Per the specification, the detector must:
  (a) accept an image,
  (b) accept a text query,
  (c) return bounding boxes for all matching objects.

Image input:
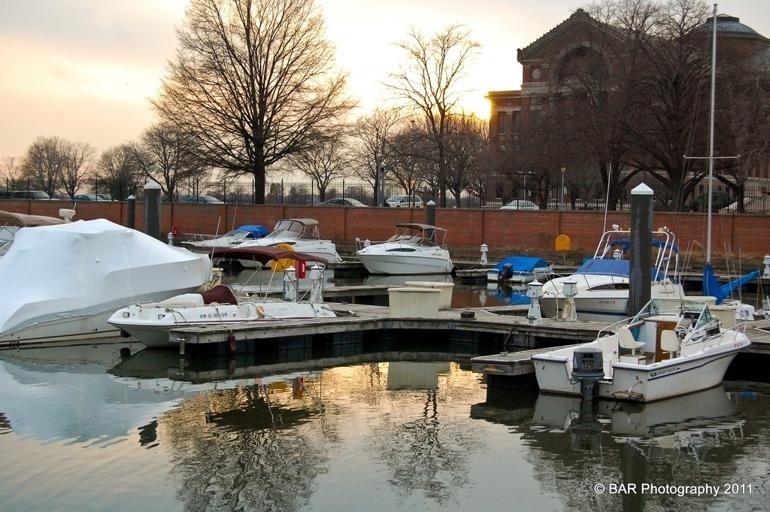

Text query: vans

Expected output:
[384,195,423,209]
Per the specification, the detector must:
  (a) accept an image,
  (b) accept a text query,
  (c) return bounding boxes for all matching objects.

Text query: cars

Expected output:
[317,197,371,208]
[178,195,226,204]
[1,190,113,201]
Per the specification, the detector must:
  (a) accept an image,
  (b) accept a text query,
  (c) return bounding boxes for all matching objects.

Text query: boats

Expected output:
[0,199,454,348]
[487,3,770,405]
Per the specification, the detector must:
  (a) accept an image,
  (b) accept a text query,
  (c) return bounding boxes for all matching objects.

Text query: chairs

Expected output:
[660,330,681,360]
[617,327,646,355]
